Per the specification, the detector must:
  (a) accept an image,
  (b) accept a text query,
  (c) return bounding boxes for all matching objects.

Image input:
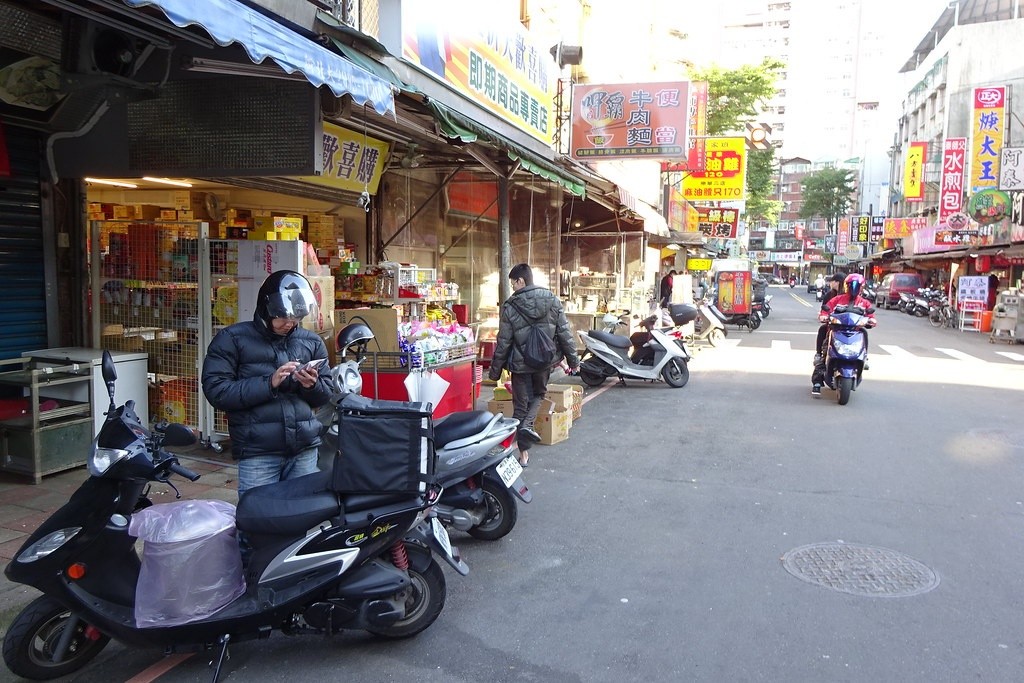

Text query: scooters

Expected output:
[631,257,774,352]
[600,295,699,355]
[313,341,533,542]
[819,304,875,406]
[814,284,828,302]
[896,285,959,329]
[576,311,694,388]
[0,350,472,683]
[789,278,796,289]
[859,279,883,304]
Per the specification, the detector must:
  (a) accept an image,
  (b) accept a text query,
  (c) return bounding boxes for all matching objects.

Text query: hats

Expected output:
[827,272,847,282]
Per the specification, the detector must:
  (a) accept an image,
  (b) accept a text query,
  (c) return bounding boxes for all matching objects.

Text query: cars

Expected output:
[758,272,784,285]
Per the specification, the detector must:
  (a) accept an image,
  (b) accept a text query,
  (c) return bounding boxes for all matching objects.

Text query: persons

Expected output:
[489,264,580,467]
[202,270,334,501]
[790,277,795,283]
[941,279,956,297]
[660,270,678,307]
[811,272,876,395]
[988,274,999,310]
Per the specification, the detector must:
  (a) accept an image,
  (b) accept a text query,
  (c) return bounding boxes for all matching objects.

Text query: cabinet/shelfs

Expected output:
[88,217,240,434]
[959,300,983,333]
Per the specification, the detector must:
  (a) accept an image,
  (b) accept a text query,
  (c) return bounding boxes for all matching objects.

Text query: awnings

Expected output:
[53,0,670,258]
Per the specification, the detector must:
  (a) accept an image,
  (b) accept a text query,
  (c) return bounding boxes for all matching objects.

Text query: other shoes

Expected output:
[864,364,869,370]
[812,386,821,395]
[814,354,821,362]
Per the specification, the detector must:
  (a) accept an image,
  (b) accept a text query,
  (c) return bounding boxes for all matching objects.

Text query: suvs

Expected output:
[874,273,925,310]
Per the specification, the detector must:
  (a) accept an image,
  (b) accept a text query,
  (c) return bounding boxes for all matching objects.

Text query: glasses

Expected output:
[511,279,518,288]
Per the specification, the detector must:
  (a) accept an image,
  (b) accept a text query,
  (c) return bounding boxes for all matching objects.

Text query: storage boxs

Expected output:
[488,382,584,445]
[84,188,458,432]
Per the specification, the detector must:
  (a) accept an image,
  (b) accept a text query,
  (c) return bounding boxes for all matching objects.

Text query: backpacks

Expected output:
[506,300,557,370]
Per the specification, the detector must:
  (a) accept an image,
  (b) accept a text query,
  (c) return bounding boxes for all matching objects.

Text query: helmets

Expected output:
[336,323,375,353]
[257,269,314,321]
[818,274,823,278]
[845,273,864,291]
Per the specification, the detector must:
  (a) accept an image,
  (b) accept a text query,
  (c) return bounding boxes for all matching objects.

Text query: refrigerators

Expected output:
[23,347,148,453]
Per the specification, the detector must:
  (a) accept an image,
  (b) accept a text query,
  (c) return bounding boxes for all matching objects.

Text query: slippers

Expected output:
[519,457,528,467]
[520,428,541,442]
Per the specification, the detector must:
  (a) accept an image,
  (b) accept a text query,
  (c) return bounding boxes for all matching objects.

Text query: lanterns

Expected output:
[975,255,991,272]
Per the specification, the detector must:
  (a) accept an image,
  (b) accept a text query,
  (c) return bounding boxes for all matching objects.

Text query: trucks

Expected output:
[804,261,833,293]
